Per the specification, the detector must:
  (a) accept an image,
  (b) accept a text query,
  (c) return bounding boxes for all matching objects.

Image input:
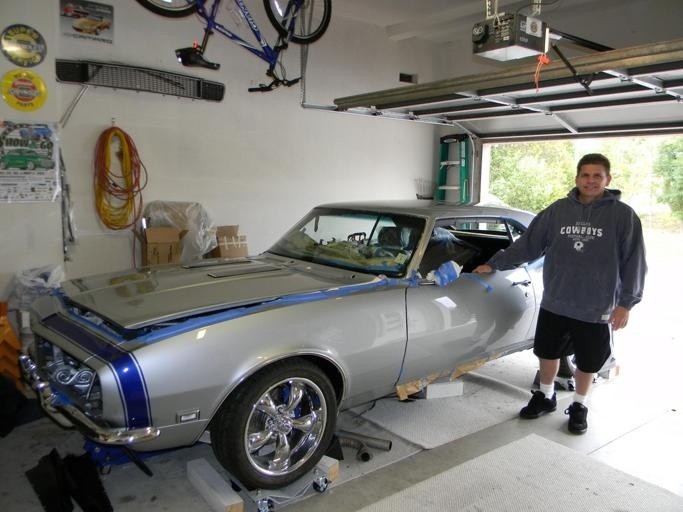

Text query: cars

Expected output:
[0,149,54,171]
[60,3,90,18]
[72,14,112,36]
[15,203,621,491]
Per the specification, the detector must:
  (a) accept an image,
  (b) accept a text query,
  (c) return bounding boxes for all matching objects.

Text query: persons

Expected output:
[470,153,647,437]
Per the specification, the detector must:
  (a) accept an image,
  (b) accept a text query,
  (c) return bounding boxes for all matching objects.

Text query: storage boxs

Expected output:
[210,224,248,257]
[130,226,193,266]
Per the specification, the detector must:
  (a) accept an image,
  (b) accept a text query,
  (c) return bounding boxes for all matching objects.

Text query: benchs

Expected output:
[368,226,511,277]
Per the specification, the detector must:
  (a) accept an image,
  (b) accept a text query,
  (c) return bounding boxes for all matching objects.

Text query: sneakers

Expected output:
[520,390,556,419]
[565,402,588,433]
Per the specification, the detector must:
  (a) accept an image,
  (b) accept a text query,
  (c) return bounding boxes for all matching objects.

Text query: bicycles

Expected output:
[137,0,337,94]
